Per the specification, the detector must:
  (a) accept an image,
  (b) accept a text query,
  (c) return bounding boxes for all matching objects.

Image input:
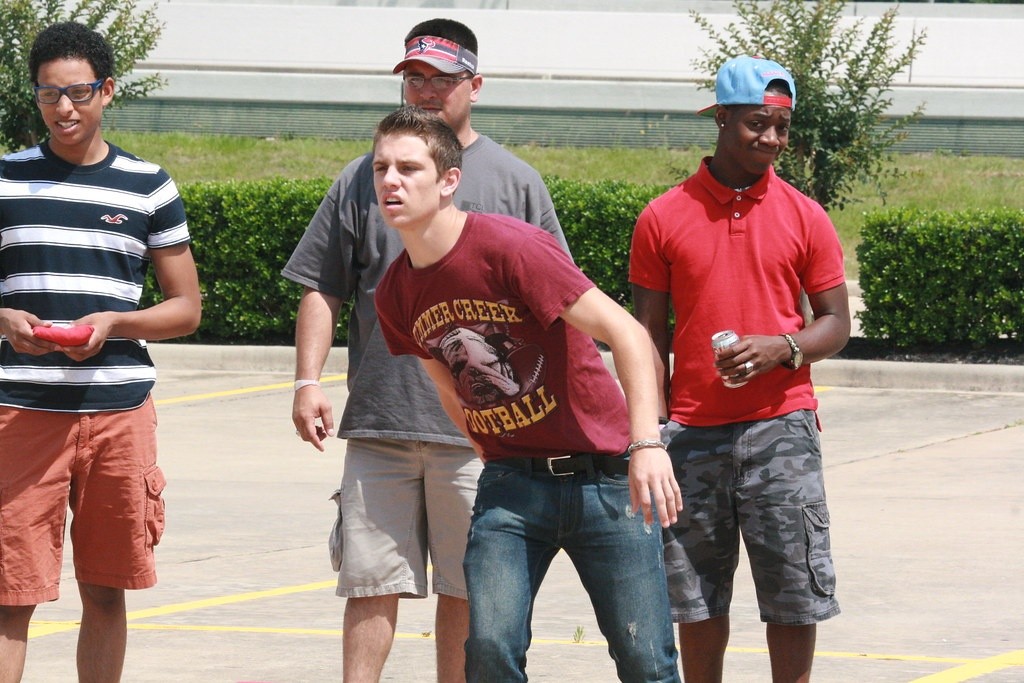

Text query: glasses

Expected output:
[402,74,471,91]
[31,80,101,104]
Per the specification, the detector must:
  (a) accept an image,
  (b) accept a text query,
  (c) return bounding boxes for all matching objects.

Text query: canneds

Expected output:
[711,330,749,388]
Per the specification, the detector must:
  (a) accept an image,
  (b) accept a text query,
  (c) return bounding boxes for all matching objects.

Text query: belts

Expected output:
[502,452,630,477]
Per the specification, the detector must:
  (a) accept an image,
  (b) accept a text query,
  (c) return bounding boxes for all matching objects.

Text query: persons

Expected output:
[0,21,200,683]
[280,19,574,683]
[628,55,857,683]
[371,107,686,683]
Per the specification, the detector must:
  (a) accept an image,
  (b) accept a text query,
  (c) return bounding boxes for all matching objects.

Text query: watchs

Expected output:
[778,332,804,370]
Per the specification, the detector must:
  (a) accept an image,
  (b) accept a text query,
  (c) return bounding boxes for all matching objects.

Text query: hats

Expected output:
[696,54,795,116]
[393,36,478,76]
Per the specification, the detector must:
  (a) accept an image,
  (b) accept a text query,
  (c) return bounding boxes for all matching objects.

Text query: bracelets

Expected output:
[293,380,320,391]
[628,440,667,453]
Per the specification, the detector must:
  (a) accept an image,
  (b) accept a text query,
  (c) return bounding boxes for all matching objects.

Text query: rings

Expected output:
[296,431,301,436]
[745,361,753,374]
